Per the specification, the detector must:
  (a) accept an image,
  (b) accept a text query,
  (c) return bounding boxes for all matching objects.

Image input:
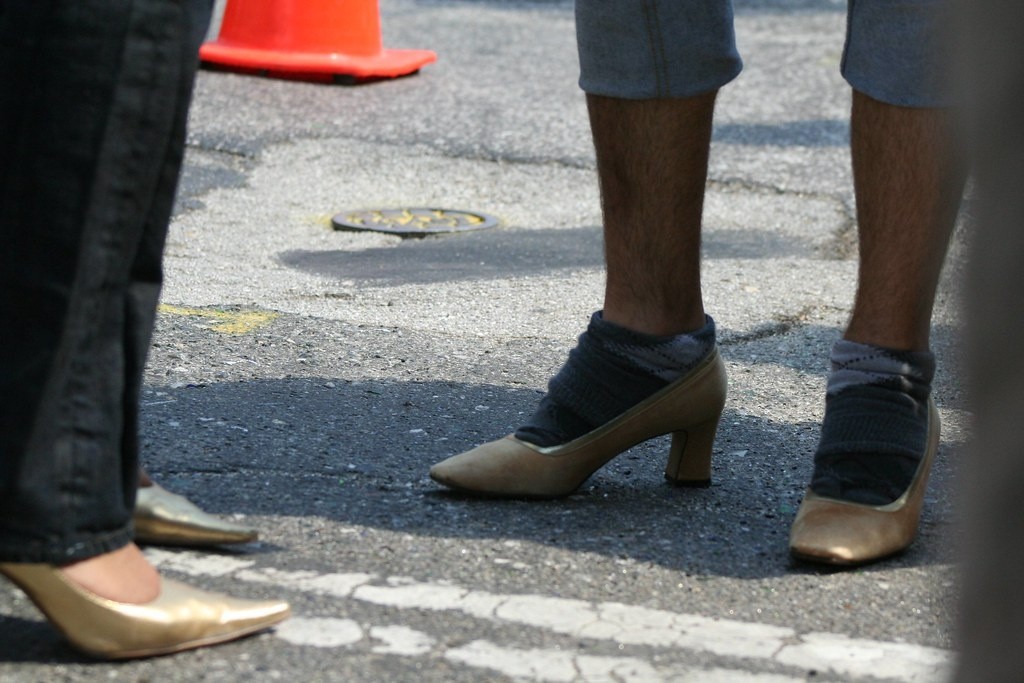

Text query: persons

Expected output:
[431,0,978,567]
[0,0,290,658]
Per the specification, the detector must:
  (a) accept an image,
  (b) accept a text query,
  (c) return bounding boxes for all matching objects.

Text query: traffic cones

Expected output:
[193,0,438,83]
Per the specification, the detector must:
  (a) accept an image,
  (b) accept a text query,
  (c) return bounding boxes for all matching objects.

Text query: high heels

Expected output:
[0,562,291,658]
[428,342,728,495]
[133,480,258,544]
[788,393,942,563]
